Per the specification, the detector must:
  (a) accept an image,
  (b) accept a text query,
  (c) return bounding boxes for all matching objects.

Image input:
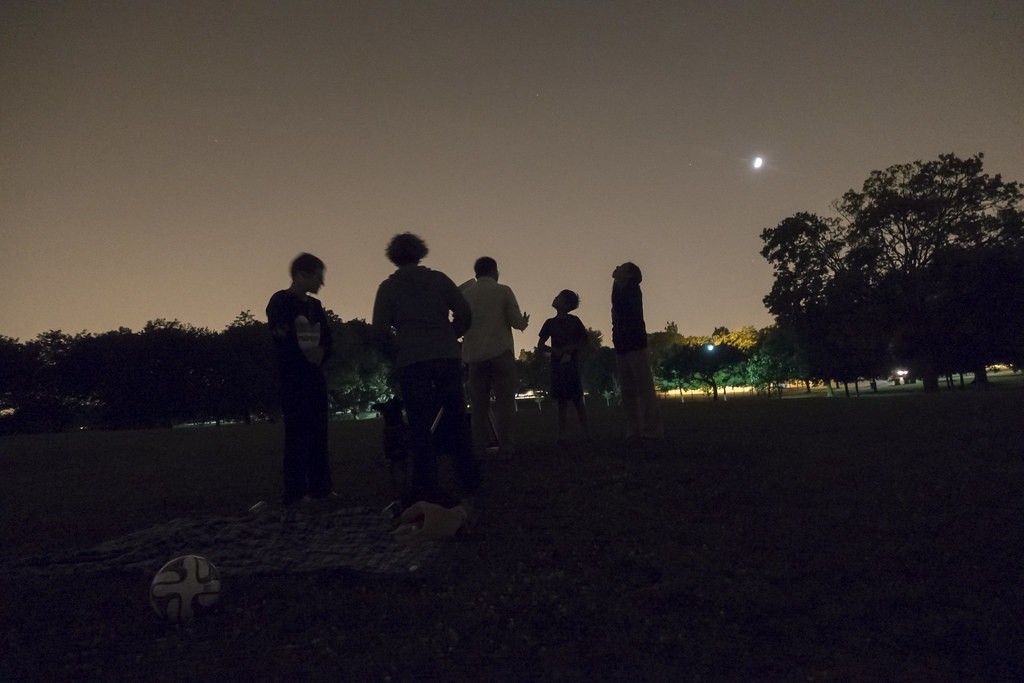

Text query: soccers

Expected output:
[149,556,223,621]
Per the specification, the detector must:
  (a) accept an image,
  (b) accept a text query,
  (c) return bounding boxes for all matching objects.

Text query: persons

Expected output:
[536,290,596,443]
[609,261,659,438]
[371,231,475,490]
[455,254,530,462]
[265,252,340,510]
